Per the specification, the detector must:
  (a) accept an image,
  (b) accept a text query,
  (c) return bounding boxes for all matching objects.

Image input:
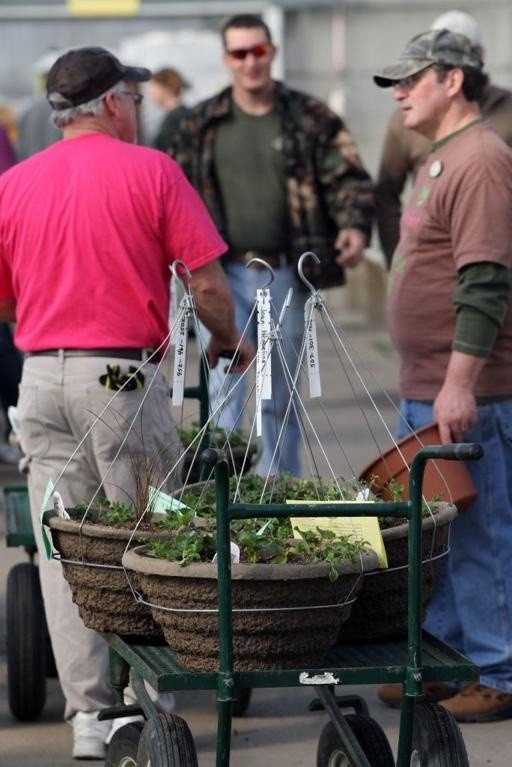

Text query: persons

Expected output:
[154,14,374,481]
[0,123,24,463]
[0,45,255,761]
[145,70,191,143]
[373,29,512,724]
[374,11,512,271]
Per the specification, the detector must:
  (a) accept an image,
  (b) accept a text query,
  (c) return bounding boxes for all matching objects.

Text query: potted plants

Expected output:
[330,476,460,644]
[171,470,327,520]
[44,502,169,640]
[172,416,259,486]
[121,510,376,675]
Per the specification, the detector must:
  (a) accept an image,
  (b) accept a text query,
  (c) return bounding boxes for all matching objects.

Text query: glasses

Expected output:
[223,42,274,61]
[99,84,146,105]
[389,65,455,89]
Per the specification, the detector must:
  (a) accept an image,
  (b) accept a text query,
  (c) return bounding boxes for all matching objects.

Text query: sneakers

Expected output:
[377,681,512,723]
[71,713,147,761]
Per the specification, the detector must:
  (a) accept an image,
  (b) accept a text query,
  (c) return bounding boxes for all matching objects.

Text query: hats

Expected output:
[432,11,482,53]
[371,28,484,90]
[41,44,154,110]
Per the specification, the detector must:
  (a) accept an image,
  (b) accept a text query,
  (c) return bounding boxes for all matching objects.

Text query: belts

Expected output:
[224,247,297,270]
[27,338,162,370]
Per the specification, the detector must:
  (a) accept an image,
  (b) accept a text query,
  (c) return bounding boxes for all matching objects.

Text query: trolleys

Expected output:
[5,436,265,723]
[47,440,491,767]
